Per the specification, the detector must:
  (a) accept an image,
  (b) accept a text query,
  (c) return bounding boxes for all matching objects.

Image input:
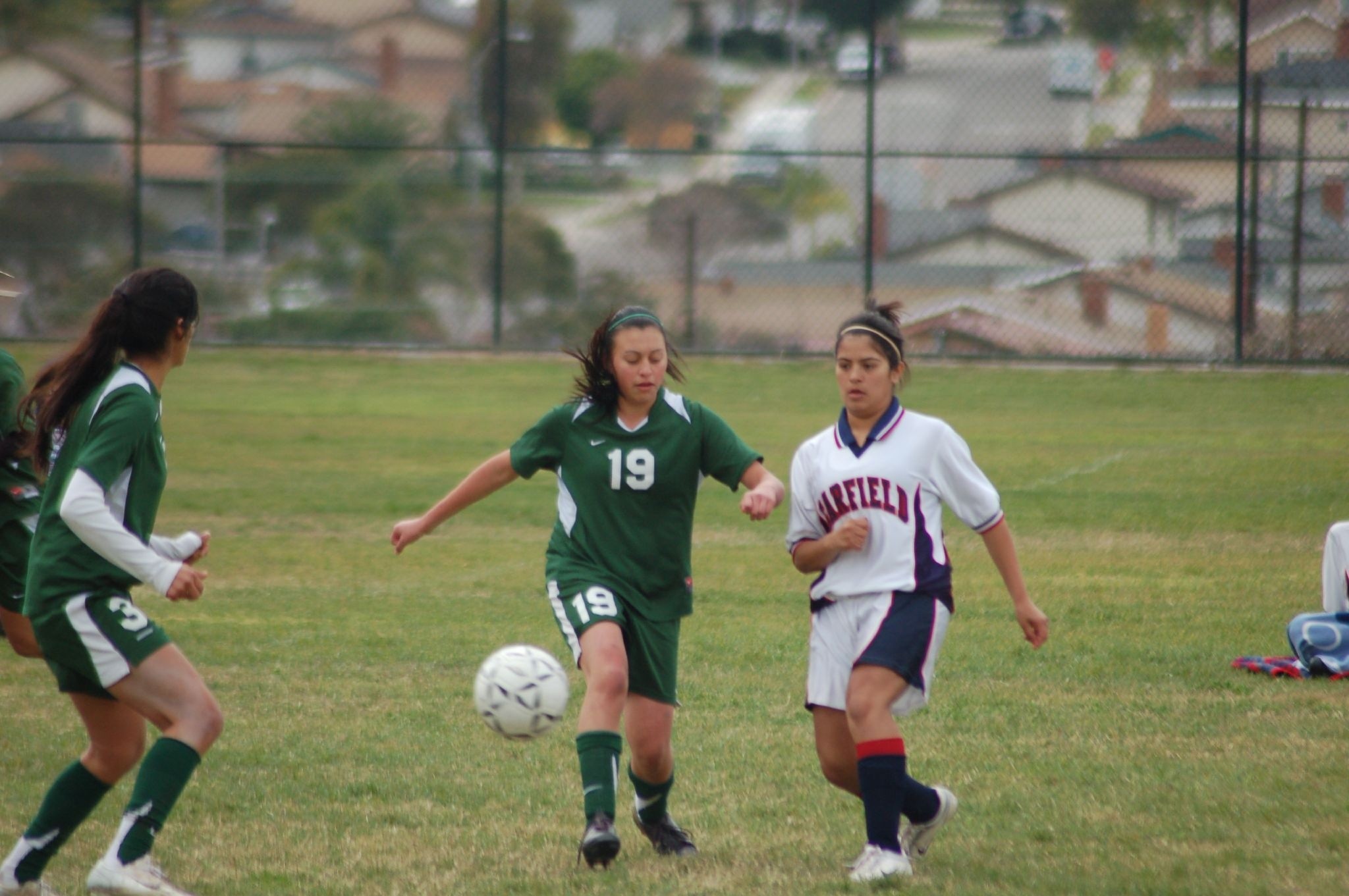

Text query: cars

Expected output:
[831,33,886,85]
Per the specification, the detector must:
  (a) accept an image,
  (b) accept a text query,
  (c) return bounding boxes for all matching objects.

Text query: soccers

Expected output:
[474,644,569,736]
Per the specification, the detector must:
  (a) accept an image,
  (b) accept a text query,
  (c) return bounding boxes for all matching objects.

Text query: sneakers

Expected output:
[86,854,192,896]
[632,806,696,862]
[899,787,958,864]
[0,881,56,896]
[846,843,915,885]
[578,812,620,869]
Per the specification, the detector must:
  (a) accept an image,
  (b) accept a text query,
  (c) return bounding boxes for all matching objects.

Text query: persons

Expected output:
[785,298,1049,882]
[0,349,45,659]
[390,307,785,872]
[0,268,225,893]
